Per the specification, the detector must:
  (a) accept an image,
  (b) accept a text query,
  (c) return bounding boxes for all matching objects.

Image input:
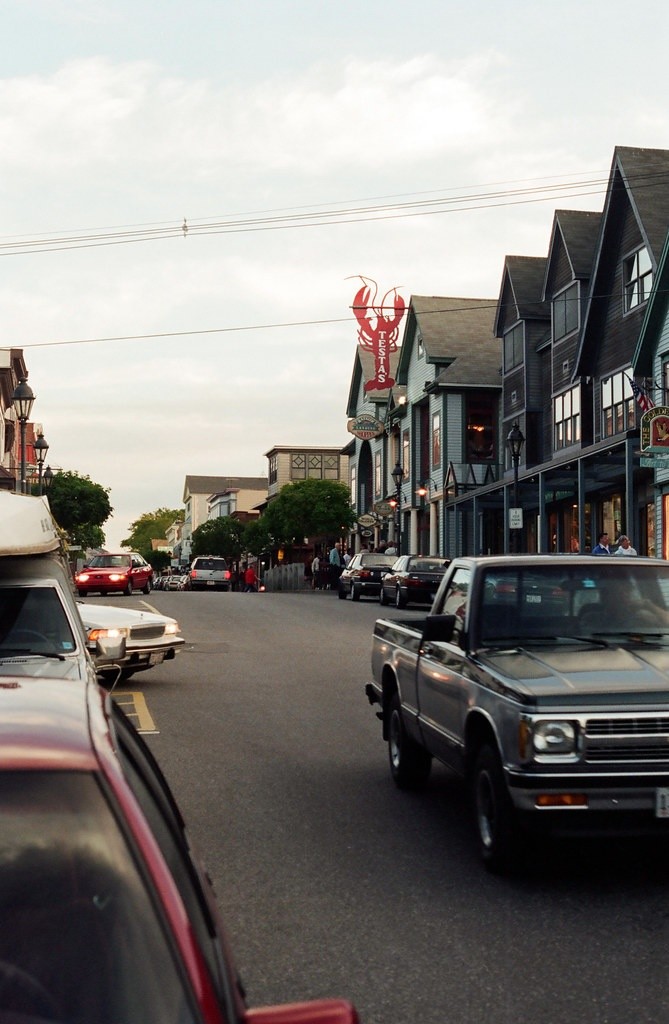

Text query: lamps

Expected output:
[415,476,438,496]
[388,490,407,507]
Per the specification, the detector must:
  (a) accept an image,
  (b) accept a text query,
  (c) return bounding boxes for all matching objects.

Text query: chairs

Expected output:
[482,604,523,635]
[573,603,604,631]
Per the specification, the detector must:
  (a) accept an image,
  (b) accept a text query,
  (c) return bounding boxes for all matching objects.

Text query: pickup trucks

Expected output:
[365,552,669,875]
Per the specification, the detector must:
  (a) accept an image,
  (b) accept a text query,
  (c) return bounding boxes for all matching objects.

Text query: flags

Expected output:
[629,377,654,412]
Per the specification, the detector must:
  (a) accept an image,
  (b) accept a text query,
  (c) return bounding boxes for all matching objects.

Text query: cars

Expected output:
[381,553,451,610]
[153,557,232,591]
[0,660,231,1024]
[0,557,89,687]
[338,553,398,601]
[75,601,187,683]
[75,551,153,596]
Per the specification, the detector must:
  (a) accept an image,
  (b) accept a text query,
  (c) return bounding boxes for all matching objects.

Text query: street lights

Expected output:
[33,430,50,497]
[390,459,405,557]
[507,418,527,551]
[10,373,37,494]
[261,560,266,593]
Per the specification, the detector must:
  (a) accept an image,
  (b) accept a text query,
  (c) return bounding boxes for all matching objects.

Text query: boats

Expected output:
[0,491,60,556]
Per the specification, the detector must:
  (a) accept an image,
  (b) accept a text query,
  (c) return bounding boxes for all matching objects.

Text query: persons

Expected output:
[230,564,260,592]
[311,553,323,590]
[361,544,369,553]
[330,543,352,590]
[615,536,637,555]
[373,540,397,554]
[592,533,611,554]
[578,574,669,627]
